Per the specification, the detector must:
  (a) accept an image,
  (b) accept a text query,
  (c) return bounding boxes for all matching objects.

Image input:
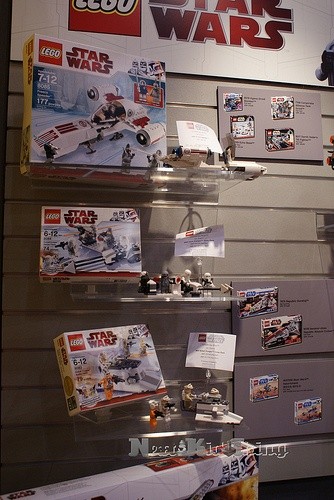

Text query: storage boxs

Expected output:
[51,323,169,419]
[19,33,167,179]
[40,206,143,285]
[0,438,259,500]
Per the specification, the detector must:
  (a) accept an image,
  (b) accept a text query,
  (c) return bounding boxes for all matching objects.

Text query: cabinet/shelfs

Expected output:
[109,165,244,439]
